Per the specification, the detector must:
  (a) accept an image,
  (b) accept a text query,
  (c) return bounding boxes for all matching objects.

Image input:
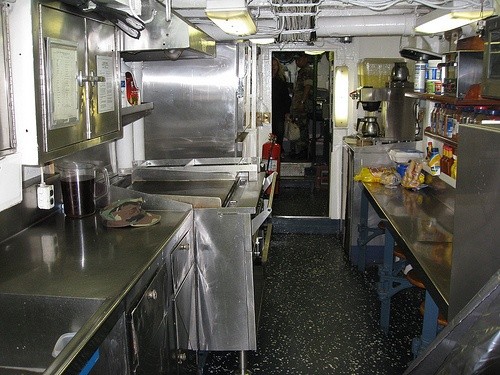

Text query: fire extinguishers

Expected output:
[262,133,280,195]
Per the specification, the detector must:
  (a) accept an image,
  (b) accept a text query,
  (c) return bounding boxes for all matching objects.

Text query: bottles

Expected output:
[121,58,141,107]
[413,57,442,95]
[429,102,500,142]
[426,141,458,180]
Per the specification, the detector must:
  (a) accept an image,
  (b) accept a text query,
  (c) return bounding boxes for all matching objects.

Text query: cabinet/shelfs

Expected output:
[404,91,500,149]
[8,0,123,167]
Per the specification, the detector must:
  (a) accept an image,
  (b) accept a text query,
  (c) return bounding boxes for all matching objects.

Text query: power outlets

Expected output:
[40,232,61,264]
[37,184,55,210]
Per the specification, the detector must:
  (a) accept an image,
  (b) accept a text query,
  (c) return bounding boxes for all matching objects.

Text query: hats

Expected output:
[99,197,161,228]
[292,52,306,59]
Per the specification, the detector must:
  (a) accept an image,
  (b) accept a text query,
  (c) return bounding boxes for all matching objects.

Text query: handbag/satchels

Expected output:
[283,116,300,141]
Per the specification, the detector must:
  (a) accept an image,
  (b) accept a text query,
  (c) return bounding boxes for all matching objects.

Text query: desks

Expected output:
[357,182,456,359]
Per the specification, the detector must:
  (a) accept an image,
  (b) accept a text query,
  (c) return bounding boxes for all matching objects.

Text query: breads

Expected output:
[368,159,424,188]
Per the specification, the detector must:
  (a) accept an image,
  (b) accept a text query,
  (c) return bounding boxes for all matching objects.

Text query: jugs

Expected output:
[356,116,380,137]
[53,161,110,219]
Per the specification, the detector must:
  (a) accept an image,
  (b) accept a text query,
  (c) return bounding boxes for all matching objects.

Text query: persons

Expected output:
[288,52,315,159]
[272,59,291,159]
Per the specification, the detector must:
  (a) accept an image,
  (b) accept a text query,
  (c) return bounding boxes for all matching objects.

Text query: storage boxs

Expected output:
[388,147,426,164]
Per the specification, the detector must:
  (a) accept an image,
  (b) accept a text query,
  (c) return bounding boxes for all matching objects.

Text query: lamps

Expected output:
[412,0,496,34]
[203,0,259,37]
[334,65,349,130]
